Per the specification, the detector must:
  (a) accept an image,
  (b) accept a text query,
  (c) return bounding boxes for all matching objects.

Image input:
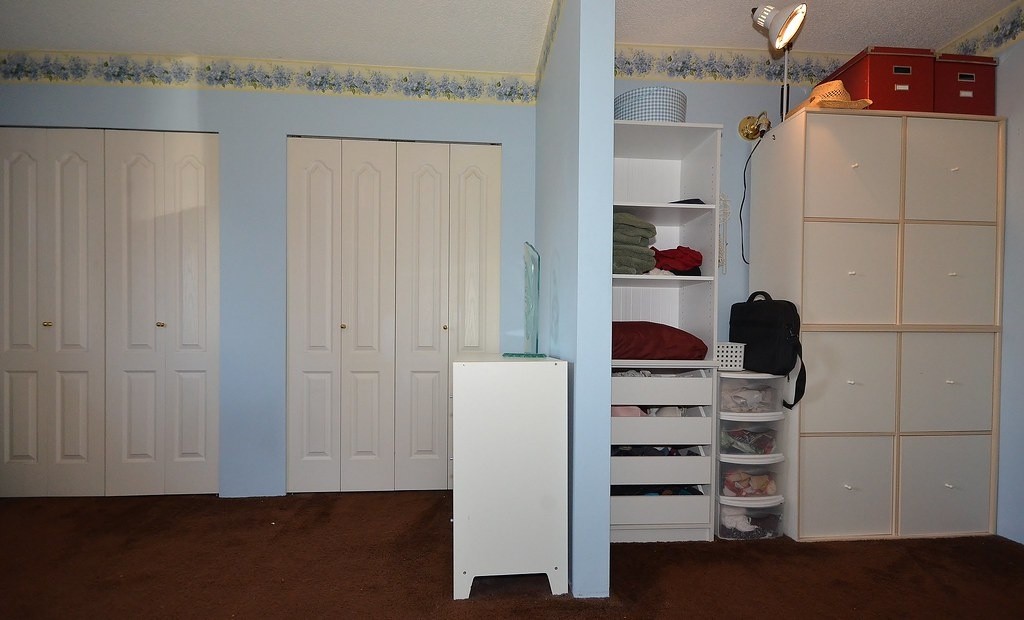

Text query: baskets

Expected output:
[716,341,747,371]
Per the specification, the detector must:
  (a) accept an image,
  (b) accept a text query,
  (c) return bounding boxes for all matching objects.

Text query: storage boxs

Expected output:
[812,45,937,113]
[933,52,998,116]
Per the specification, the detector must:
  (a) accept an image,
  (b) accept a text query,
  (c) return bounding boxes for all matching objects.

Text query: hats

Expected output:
[807,80,873,109]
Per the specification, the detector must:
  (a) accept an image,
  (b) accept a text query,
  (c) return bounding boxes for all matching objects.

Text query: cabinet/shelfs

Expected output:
[452,353,568,602]
[608,119,717,544]
[749,107,994,540]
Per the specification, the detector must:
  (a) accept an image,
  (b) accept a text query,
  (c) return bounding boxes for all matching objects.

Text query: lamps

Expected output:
[749,2,808,124]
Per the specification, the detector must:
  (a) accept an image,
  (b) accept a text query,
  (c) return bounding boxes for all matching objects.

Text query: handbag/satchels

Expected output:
[728,291,806,409]
